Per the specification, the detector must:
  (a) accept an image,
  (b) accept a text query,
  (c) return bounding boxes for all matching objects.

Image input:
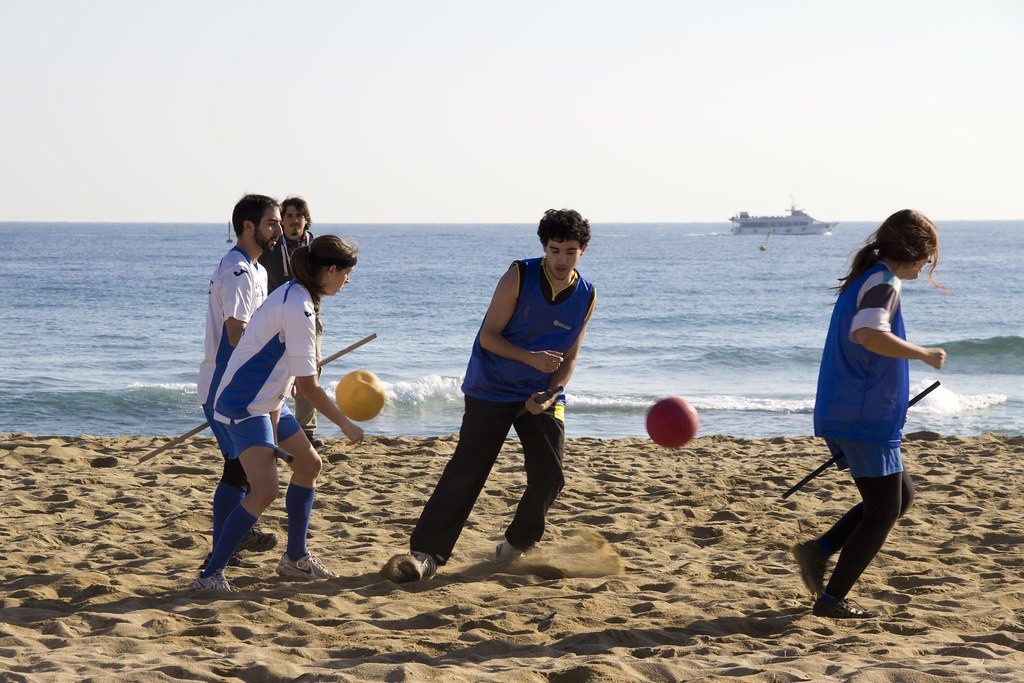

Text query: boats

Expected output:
[728,207,839,235]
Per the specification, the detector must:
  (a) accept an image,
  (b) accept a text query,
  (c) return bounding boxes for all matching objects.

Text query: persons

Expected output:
[398,209,598,582]
[196,194,284,571]
[191,234,364,592]
[792,208,948,620]
[257,196,325,449]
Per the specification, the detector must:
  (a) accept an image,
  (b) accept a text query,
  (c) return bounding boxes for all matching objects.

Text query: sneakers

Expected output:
[397,551,437,579]
[495,542,526,562]
[812,594,881,619]
[204,552,260,569]
[191,568,235,593]
[275,549,340,580]
[235,528,278,553]
[791,540,829,599]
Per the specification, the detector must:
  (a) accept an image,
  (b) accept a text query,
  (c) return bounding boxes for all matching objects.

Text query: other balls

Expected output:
[337,371,384,419]
[646,397,700,445]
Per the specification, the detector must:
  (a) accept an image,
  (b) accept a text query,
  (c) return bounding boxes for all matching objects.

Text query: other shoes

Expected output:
[305,435,324,448]
